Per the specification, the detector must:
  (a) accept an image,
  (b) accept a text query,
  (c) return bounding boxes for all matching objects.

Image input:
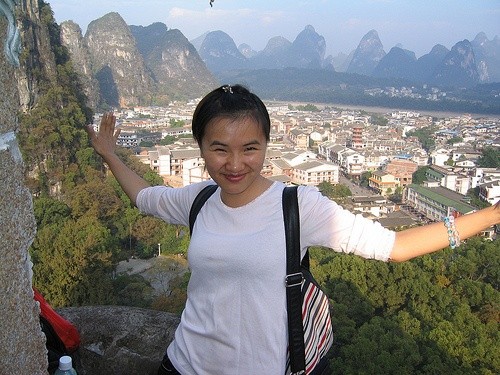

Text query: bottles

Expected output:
[55,356,77,375]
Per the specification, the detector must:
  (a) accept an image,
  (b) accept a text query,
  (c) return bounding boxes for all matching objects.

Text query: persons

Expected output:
[88,85,500,375]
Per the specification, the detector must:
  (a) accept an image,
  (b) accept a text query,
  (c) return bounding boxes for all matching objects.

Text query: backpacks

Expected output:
[188,183,333,375]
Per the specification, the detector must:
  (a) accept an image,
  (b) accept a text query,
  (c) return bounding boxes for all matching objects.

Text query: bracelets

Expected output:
[444,217,456,250]
[450,216,460,250]
